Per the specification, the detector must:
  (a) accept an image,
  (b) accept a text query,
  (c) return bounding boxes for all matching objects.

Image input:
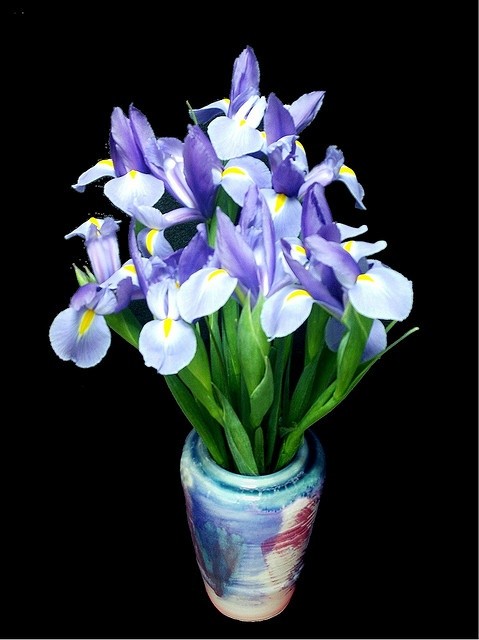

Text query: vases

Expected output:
[179,429,324,625]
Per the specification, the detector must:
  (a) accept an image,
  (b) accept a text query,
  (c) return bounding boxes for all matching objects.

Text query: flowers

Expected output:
[49,44,420,474]
